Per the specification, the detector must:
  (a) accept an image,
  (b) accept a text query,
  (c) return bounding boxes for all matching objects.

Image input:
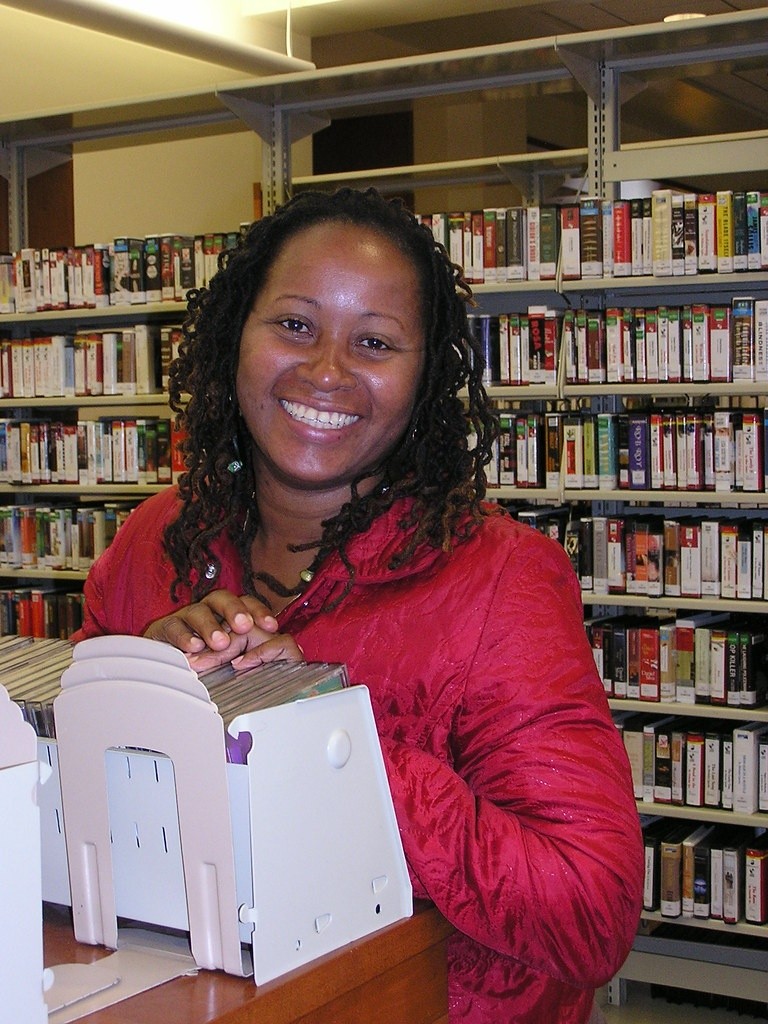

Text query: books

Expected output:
[0,634,350,762]
[0,223,250,640]
[416,188,768,925]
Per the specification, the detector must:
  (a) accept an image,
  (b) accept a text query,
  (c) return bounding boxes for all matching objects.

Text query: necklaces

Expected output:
[242,490,302,618]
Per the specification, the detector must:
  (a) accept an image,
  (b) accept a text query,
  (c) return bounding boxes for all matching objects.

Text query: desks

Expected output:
[41,897,456,1024]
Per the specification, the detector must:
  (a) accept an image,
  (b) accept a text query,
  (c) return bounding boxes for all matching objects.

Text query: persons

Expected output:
[65,190,644,1024]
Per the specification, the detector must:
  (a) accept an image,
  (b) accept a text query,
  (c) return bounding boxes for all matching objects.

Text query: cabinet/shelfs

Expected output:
[1,6,768,1007]
